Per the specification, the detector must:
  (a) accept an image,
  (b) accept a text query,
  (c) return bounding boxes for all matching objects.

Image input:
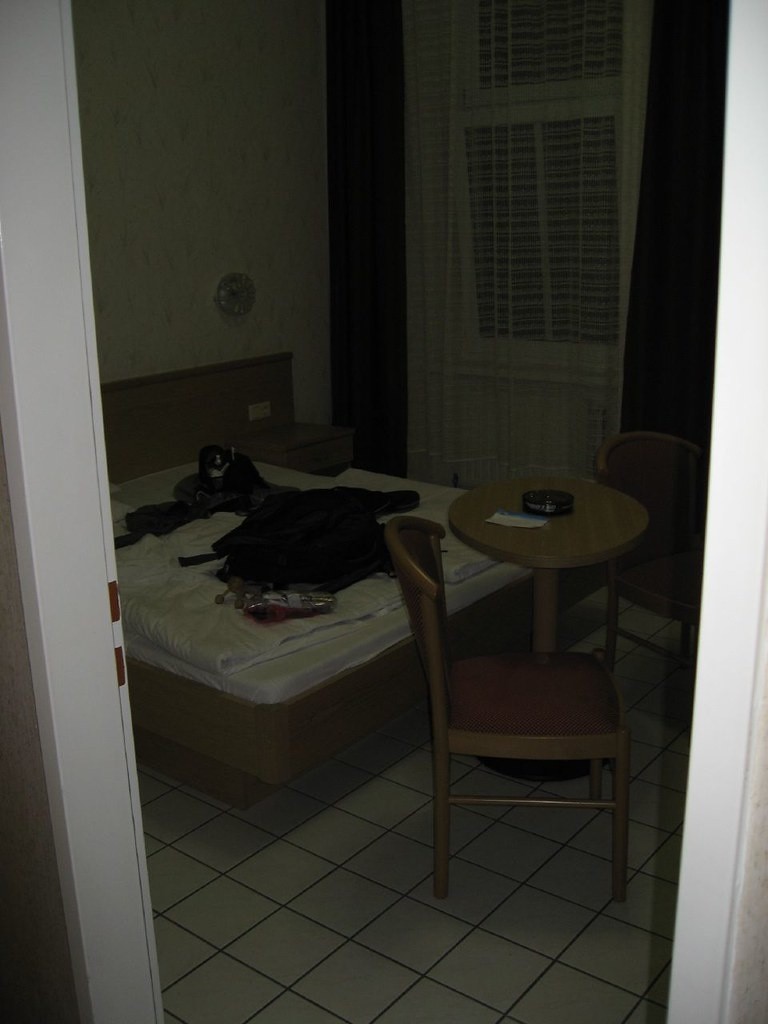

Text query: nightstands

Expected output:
[224,421,354,476]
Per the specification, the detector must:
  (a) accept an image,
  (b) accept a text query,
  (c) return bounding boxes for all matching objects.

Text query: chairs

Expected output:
[386,517,628,902]
[596,432,709,678]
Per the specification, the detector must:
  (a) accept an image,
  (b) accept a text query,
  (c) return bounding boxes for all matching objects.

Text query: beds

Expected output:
[99,352,602,786]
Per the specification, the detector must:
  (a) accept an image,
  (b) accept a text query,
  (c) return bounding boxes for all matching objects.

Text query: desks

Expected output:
[449,477,648,783]
